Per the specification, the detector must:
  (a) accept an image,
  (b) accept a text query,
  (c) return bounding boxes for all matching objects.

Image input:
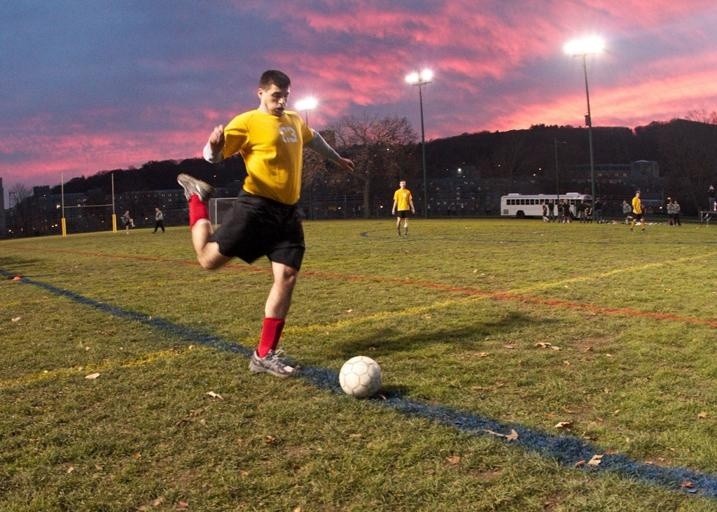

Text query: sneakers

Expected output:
[248,347,298,378]
[176,173,214,202]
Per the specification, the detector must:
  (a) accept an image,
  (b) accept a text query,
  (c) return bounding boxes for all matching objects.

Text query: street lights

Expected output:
[559,29,609,221]
[293,95,319,220]
[403,67,436,218]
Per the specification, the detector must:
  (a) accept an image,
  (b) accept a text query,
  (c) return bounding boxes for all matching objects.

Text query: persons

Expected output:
[120,209,135,235]
[628,190,645,232]
[390,179,416,238]
[175,67,358,379]
[540,196,681,226]
[151,207,166,234]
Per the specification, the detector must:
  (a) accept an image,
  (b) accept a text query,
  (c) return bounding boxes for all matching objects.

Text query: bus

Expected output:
[499,191,592,219]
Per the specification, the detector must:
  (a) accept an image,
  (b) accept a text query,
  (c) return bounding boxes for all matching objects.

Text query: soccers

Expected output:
[338,355,382,399]
